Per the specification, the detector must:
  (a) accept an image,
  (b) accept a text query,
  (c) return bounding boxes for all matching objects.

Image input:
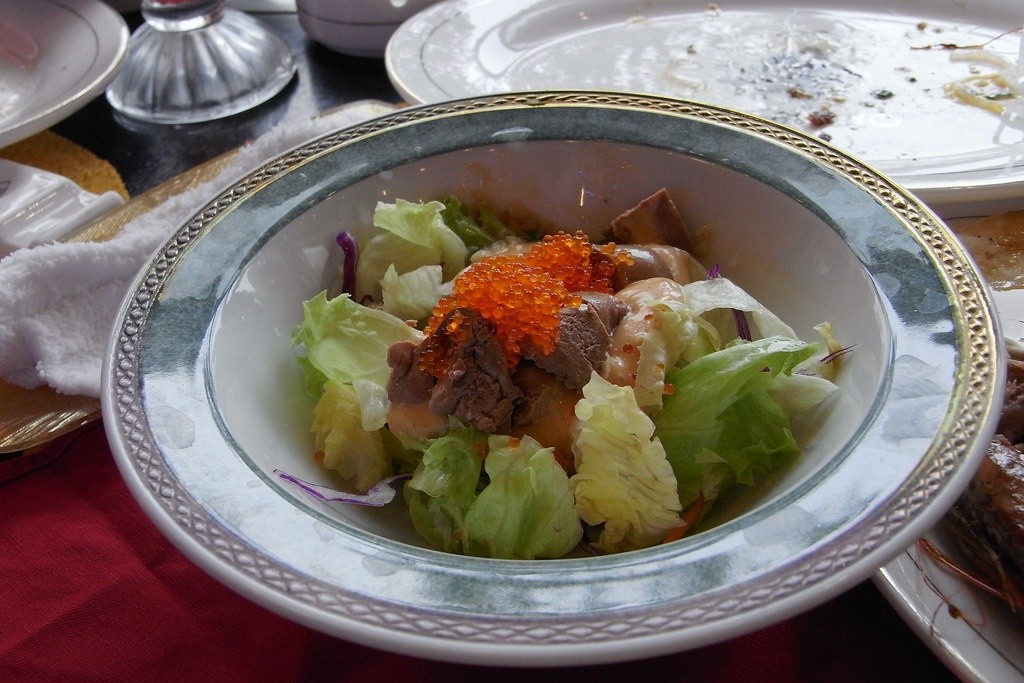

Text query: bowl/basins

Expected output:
[97,89,1008,670]
[295,1,438,57]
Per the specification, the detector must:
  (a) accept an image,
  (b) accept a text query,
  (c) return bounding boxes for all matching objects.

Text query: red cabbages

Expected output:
[274,467,413,508]
[335,230,359,304]
[707,263,751,340]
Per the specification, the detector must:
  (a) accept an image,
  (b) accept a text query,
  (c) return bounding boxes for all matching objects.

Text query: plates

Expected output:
[875,526,1024,683]
[382,0,1023,213]
[0,1,132,151]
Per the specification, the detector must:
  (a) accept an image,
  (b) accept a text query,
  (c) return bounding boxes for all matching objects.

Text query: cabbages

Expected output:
[289,195,843,561]
